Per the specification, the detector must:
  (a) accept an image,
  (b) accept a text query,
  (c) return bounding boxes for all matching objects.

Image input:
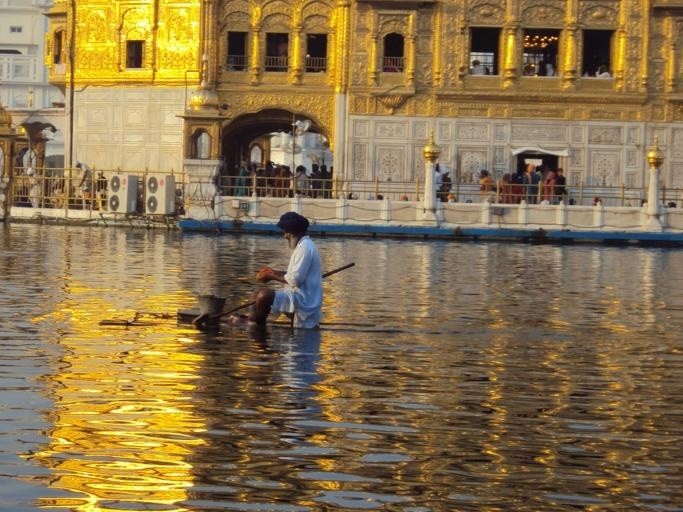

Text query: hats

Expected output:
[277,213,309,235]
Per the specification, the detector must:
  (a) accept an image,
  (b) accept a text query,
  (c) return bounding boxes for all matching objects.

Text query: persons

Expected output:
[434,162,456,202]
[232,157,332,196]
[75,161,108,209]
[469,53,613,78]
[480,161,566,203]
[248,212,322,328]
[175,188,183,214]
[27,168,42,209]
